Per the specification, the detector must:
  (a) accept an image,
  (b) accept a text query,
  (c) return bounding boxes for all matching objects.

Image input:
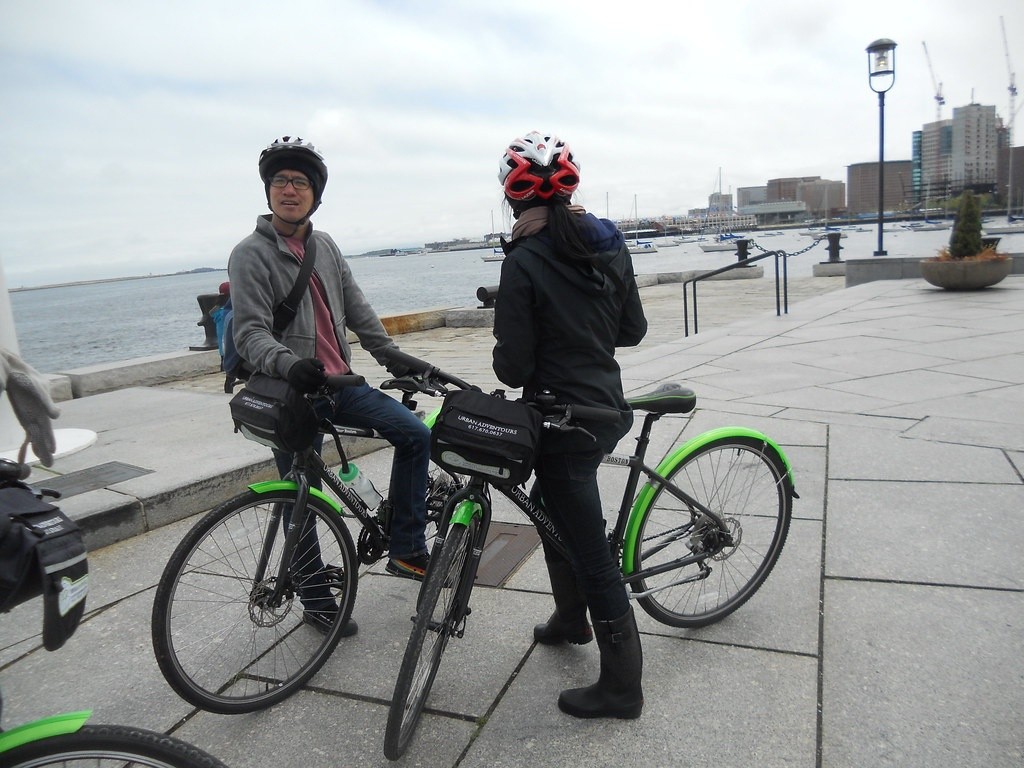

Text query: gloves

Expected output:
[402,364,445,393]
[4,371,61,467]
[287,358,324,393]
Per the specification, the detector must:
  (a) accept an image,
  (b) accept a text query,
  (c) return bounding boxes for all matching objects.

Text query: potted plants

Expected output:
[919,191,1013,289]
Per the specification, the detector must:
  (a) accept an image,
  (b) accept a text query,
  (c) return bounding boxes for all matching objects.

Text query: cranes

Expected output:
[999,16,1023,147]
[922,40,946,121]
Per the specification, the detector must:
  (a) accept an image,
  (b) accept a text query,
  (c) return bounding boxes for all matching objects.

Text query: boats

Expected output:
[855,228,872,232]
[418,250,426,255]
[756,232,785,237]
[843,226,856,230]
[396,251,408,256]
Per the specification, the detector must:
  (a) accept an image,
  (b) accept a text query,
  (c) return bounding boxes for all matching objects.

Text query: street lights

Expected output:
[867,38,897,255]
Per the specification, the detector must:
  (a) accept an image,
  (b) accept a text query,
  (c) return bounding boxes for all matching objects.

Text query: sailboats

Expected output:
[606,192,657,254]
[799,180,848,238]
[698,166,755,251]
[481,209,506,261]
[884,145,1024,234]
[656,221,709,247]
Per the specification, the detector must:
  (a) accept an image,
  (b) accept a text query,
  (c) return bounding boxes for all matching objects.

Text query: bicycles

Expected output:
[0,710,230,768]
[150,349,471,715]
[382,345,800,762]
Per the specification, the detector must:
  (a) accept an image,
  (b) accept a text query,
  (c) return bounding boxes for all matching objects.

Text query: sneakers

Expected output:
[385,551,453,588]
[302,603,358,639]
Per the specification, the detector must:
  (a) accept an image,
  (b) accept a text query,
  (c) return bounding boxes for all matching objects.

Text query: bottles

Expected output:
[339,463,383,511]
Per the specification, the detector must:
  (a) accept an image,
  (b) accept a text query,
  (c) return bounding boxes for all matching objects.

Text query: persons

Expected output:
[494,130,648,721]
[227,136,451,636]
[214,282,231,356]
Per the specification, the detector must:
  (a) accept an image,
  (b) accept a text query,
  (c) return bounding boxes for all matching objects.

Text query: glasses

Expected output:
[268,176,314,190]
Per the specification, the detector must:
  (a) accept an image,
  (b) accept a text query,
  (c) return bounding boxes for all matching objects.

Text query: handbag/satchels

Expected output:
[209,228,316,394]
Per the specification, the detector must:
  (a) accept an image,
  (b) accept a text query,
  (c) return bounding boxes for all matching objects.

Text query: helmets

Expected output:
[258,134,329,199]
[498,130,581,203]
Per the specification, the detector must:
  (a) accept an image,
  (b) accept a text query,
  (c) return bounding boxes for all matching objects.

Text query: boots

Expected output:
[558,604,644,720]
[533,555,593,646]
